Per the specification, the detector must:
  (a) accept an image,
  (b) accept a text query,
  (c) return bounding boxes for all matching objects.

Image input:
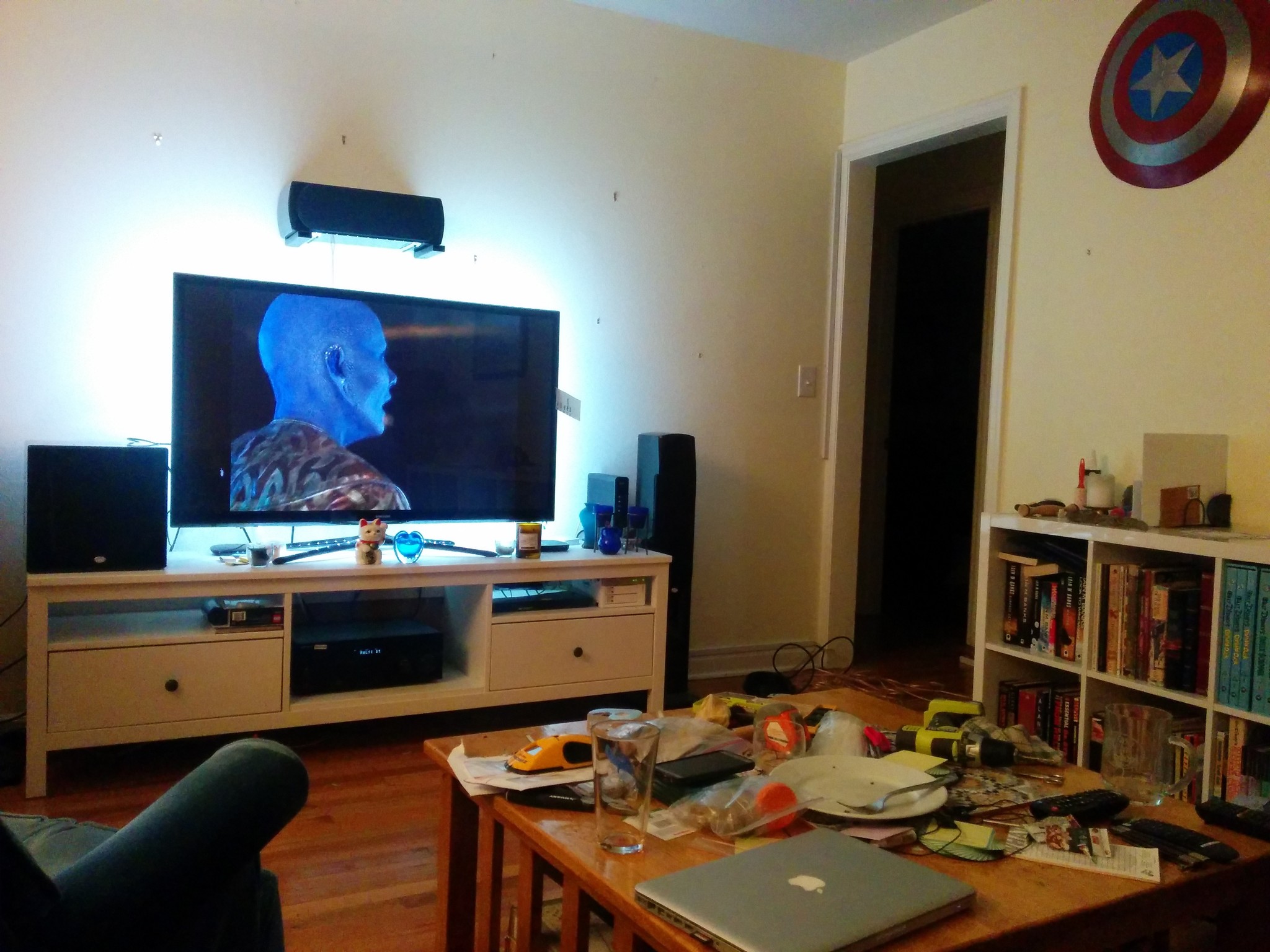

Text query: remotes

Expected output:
[1124,817,1240,865]
[1194,795,1270,842]
[1029,787,1129,825]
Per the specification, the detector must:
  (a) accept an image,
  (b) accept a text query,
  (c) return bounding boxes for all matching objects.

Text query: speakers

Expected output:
[22,443,166,571]
[634,431,698,709]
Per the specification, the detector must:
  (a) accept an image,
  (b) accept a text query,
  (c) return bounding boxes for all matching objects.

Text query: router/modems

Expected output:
[540,539,569,553]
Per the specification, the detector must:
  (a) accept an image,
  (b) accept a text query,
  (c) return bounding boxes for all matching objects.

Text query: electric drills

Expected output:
[891,696,1055,770]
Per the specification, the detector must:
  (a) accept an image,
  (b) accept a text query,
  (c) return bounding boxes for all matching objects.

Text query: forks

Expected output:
[836,773,958,816]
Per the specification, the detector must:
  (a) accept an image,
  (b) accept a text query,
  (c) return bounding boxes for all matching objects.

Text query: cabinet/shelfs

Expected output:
[971,508,1269,814]
[24,541,674,801]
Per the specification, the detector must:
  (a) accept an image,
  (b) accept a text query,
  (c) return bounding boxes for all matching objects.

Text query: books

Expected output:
[997,537,1270,816]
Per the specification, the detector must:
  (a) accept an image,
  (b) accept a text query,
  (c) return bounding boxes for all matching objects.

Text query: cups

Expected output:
[589,719,661,854]
[246,543,272,567]
[1100,703,1198,807]
[495,540,515,558]
[516,523,542,559]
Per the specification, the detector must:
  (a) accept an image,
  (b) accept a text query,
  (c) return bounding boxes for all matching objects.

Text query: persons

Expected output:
[225,293,413,511]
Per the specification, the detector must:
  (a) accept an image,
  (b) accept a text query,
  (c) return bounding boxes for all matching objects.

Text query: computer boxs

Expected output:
[586,473,628,536]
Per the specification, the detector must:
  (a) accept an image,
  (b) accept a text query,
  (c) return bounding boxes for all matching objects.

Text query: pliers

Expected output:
[497,787,639,817]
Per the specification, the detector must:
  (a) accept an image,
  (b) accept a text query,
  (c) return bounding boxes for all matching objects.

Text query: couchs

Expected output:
[0,736,312,952]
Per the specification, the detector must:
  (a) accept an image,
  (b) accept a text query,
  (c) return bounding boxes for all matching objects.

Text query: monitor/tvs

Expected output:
[168,272,563,523]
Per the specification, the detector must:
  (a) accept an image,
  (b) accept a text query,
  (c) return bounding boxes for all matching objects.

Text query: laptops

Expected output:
[634,828,975,952]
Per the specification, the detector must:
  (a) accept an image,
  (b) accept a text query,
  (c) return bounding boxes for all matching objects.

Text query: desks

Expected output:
[424,686,1270,952]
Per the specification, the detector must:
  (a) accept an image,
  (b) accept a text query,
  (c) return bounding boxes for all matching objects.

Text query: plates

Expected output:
[768,755,948,819]
